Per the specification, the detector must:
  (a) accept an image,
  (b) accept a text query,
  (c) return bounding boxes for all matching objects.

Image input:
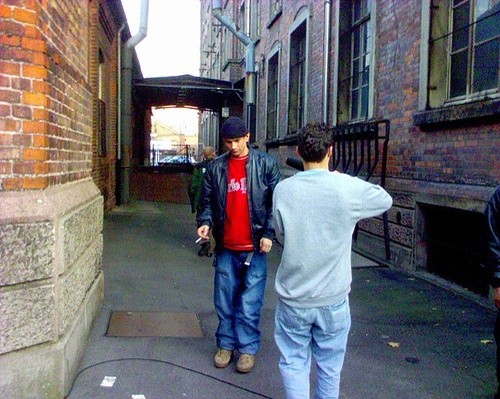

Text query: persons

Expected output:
[196,116,283,372]
[483,185,500,399]
[188,146,217,256]
[272,124,392,399]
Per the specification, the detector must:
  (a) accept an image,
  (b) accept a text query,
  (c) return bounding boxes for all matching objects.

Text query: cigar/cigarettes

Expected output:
[196,237,202,243]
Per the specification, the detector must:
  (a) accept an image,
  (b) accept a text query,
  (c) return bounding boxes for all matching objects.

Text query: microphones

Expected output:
[286,158,304,171]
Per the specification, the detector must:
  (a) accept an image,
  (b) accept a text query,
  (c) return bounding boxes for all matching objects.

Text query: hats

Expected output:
[221,117,248,137]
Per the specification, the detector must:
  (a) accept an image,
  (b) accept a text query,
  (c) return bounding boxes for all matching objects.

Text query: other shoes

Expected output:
[214,348,231,368]
[236,353,255,374]
